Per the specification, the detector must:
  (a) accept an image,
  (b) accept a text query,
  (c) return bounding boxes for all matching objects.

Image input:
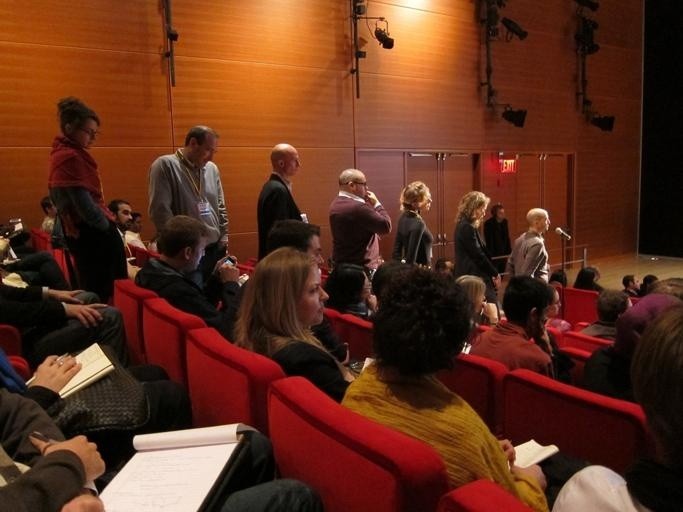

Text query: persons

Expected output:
[1,167,683,512]
[47,95,129,304]
[146,124,230,291]
[256,142,304,259]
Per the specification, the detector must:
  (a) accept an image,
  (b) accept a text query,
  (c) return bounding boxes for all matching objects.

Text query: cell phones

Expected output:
[221,256,235,268]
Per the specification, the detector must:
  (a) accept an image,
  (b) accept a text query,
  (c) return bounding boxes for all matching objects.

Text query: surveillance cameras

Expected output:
[354,0,369,16]
[500,16,528,40]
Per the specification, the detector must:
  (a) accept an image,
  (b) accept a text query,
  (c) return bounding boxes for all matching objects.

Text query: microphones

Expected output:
[555,227,572,241]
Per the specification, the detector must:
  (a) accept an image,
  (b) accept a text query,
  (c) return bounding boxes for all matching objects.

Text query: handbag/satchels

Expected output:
[50,345,150,439]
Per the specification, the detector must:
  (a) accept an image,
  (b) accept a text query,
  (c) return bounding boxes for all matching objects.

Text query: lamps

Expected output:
[470,0,533,131]
[155,0,180,89]
[341,0,396,98]
[566,1,617,133]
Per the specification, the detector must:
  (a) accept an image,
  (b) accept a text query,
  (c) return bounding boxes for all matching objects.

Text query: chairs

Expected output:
[0,229,682,511]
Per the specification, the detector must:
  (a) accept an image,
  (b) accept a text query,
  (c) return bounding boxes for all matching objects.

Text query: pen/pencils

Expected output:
[507,440,512,471]
[24,353,69,386]
